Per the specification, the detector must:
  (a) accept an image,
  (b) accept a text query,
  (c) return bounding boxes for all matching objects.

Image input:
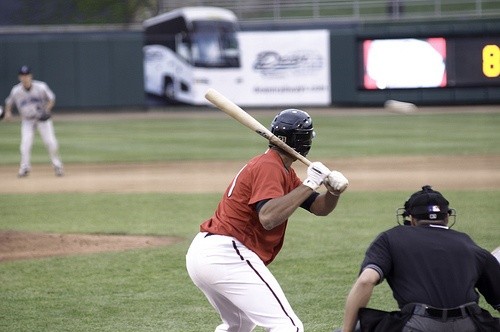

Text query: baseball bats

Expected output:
[203,88,349,193]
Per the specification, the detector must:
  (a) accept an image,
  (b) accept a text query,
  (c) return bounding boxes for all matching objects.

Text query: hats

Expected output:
[18,65,31,75]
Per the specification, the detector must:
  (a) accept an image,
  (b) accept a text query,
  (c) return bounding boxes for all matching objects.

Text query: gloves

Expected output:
[303,161,331,190]
[324,170,349,196]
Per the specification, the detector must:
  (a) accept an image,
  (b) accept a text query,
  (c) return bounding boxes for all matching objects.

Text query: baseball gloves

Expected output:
[36,107,51,122]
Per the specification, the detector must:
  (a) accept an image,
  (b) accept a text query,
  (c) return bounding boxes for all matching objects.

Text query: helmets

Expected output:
[404,184,449,220]
[267,109,314,158]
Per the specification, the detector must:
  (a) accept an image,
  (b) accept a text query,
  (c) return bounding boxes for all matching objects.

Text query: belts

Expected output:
[400,302,477,318]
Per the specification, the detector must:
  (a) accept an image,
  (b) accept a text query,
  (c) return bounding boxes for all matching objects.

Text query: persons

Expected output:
[185,108,349,332]
[2,65,66,178]
[340,184,500,332]
